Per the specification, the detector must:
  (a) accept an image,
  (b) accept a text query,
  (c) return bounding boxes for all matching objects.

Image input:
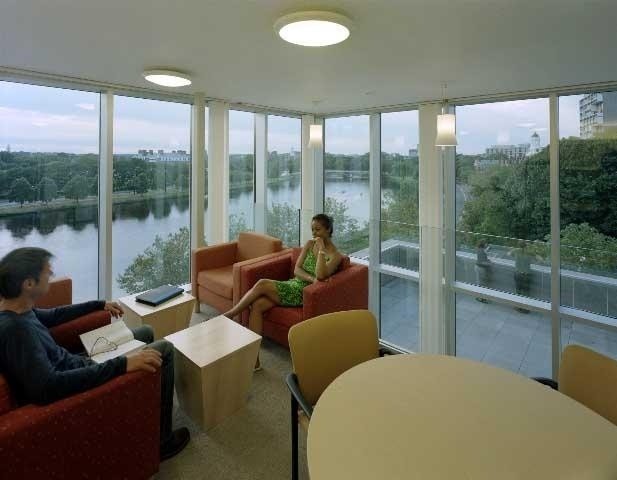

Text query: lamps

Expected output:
[433,83,458,152]
[140,69,193,89]
[270,9,353,47]
[305,113,325,150]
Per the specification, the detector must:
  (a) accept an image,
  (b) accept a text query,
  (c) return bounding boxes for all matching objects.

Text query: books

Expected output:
[79,320,146,364]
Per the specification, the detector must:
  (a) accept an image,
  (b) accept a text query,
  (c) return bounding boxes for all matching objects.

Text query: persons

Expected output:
[507,241,543,313]
[222,214,342,371]
[474,240,491,303]
[0,247,190,462]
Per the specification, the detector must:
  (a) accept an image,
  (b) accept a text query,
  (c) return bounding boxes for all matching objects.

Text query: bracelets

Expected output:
[318,251,327,254]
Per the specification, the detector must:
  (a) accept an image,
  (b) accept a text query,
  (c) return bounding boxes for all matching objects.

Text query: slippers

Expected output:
[253,365,263,372]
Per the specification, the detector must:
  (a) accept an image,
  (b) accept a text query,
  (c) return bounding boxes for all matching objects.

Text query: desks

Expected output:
[304,350,616,480]
[113,282,198,341]
[164,314,264,435]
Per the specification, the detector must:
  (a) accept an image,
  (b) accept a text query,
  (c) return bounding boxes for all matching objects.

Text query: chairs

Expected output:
[529,343,616,425]
[284,307,404,479]
[239,248,371,348]
[188,229,296,318]
[0,272,87,320]
[0,307,166,479]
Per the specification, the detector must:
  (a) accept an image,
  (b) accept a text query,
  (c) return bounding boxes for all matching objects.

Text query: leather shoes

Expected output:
[160,426,191,462]
